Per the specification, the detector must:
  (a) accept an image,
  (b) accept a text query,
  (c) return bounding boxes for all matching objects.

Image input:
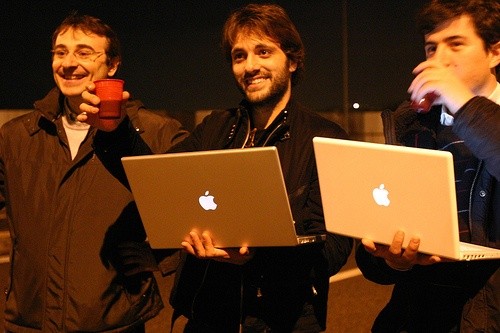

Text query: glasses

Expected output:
[50,48,104,59]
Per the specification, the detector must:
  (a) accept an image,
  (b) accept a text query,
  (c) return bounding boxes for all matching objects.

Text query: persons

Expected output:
[0,10,188,333]
[355,0,500,333]
[77,4,355,333]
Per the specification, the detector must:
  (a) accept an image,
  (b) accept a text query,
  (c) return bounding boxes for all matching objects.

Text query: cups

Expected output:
[93,79,124,119]
[409,92,438,112]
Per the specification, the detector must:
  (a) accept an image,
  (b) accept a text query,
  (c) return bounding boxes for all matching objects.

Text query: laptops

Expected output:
[121,147,327,249]
[313,136,500,261]
